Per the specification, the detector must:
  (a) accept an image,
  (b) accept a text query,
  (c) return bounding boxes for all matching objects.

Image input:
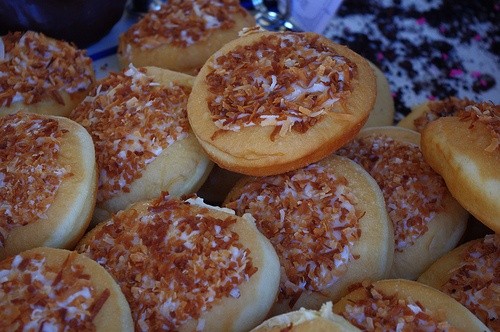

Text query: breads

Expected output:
[0,0,498,332]
